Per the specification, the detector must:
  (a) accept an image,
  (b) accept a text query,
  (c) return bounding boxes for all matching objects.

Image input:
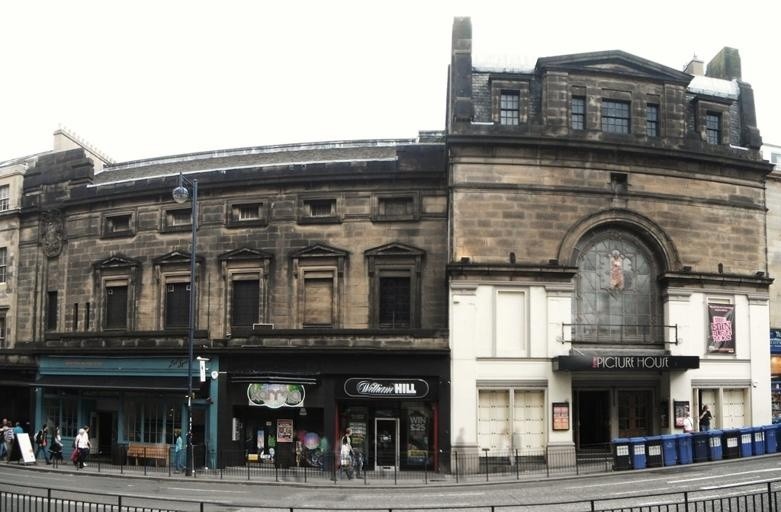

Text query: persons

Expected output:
[699,405,712,432]
[173,430,186,474]
[683,412,693,433]
[341,428,355,471]
[0,416,93,469]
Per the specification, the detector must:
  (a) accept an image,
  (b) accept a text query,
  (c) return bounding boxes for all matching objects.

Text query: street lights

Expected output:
[172,172,199,476]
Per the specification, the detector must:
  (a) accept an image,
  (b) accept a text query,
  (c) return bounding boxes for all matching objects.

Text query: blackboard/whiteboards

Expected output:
[16,432,37,463]
[8,435,20,461]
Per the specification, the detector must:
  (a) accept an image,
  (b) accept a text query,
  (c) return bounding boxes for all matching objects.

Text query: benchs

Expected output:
[125,439,168,467]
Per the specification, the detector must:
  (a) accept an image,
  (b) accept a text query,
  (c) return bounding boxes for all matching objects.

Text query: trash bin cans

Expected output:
[611,423,781,471]
